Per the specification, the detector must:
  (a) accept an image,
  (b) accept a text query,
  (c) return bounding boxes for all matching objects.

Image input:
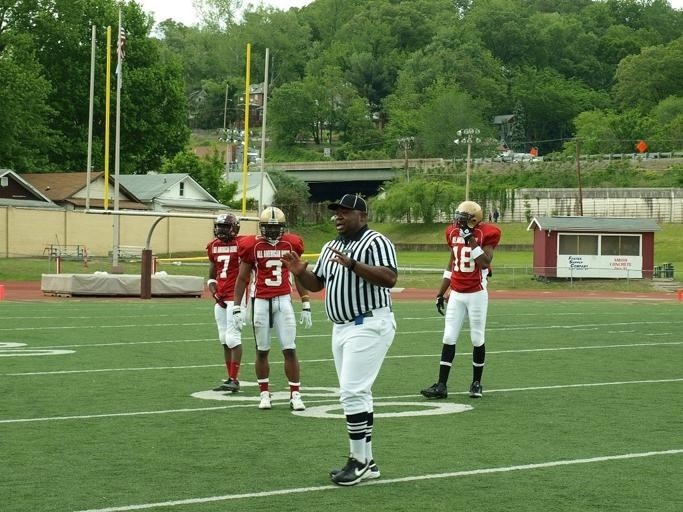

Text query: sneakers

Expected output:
[333,457,372,486]
[329,460,381,480]
[213,381,238,392]
[220,377,240,390]
[289,391,305,410]
[468,380,483,398]
[258,391,272,409]
[421,381,448,399]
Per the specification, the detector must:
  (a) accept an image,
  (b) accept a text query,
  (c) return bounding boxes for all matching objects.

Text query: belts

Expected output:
[337,306,392,325]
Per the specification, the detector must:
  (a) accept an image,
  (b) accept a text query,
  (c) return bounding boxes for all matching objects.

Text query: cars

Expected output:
[498,152,533,161]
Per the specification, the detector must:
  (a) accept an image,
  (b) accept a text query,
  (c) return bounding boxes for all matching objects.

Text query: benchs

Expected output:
[115,245,146,263]
[48,244,90,260]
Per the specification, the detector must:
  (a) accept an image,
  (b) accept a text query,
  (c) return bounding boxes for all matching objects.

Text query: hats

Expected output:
[327,192,368,212]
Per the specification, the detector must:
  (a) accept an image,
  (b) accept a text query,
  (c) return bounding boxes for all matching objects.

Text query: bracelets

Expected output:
[469,246,484,259]
[206,278,217,287]
[232,306,240,314]
[302,308,311,312]
[300,295,309,298]
[348,258,356,272]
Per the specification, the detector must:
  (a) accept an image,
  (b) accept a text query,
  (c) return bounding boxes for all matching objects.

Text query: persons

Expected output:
[205,214,250,392]
[492,209,498,223]
[279,194,397,485]
[232,206,311,410]
[419,201,501,398]
[488,212,492,223]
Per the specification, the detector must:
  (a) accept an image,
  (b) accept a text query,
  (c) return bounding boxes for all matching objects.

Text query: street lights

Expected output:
[396,136,415,224]
[215,126,244,171]
[452,128,482,203]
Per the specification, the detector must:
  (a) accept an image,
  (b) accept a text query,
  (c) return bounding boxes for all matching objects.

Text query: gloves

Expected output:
[299,301,312,330]
[233,306,243,330]
[435,293,448,316]
[458,224,474,244]
[212,291,228,309]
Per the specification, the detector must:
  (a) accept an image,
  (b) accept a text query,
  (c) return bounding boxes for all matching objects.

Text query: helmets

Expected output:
[258,205,287,239]
[212,212,240,243]
[453,199,483,230]
